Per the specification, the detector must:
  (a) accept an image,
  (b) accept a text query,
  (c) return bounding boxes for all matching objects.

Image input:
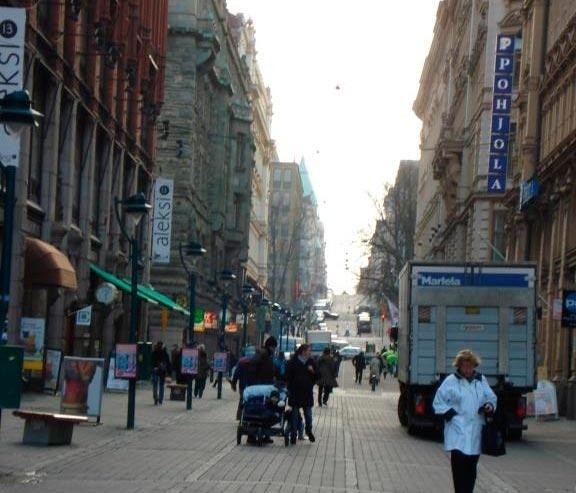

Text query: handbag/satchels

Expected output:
[482,423,505,455]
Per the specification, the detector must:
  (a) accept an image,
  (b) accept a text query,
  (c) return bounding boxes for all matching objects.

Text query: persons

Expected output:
[227,335,342,444]
[369,346,387,385]
[430,349,499,492]
[352,350,366,384]
[148,339,235,408]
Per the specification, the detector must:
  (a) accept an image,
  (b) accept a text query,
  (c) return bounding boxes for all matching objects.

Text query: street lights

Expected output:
[0,87,44,141]
[237,282,310,359]
[214,269,233,398]
[179,240,207,409]
[114,192,151,428]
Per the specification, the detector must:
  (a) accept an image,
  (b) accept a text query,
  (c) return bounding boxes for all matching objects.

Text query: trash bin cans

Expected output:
[0,344,24,408]
[138,341,152,379]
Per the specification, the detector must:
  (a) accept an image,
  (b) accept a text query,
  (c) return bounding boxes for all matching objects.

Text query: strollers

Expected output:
[236,383,294,447]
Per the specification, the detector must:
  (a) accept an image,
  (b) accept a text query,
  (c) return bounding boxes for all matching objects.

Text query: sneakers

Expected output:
[298,430,315,442]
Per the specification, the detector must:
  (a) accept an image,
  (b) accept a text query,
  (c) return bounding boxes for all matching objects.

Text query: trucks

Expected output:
[394,260,540,442]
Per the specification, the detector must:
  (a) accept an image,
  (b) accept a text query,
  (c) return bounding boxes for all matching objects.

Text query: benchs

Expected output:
[167,384,188,400]
[13,410,88,445]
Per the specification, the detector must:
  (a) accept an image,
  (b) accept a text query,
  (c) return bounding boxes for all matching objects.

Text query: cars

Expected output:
[276,302,379,358]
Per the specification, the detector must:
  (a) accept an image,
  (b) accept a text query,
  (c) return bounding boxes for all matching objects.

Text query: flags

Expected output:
[386,302,400,326]
[76,305,93,328]
